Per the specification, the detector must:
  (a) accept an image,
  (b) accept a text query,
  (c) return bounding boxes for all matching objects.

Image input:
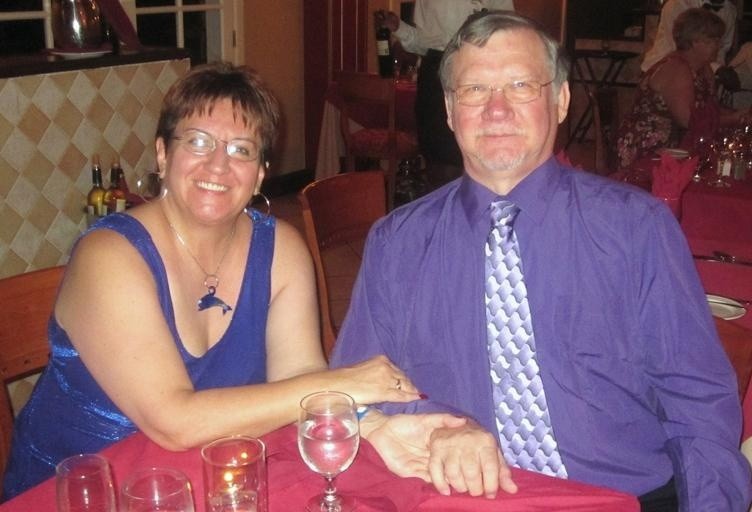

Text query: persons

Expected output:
[330,9,751,512]
[615,0,751,173]
[0,61,467,501]
[381,0,517,192]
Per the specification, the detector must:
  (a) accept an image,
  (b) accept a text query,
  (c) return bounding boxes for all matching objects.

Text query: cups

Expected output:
[56,454,117,511]
[200,435,269,512]
[120,465,195,512]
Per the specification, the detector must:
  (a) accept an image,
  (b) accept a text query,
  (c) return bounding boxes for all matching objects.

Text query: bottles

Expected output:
[377,8,394,78]
[103,156,125,216]
[87,155,105,226]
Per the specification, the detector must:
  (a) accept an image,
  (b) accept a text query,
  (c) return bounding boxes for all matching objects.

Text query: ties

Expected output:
[485,201,568,480]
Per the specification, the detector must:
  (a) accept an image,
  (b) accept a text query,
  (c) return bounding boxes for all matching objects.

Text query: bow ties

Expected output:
[702,3,723,12]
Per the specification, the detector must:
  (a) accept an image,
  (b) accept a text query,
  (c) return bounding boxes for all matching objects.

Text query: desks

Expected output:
[0,417,641,512]
[564,46,639,150]
[323,76,418,129]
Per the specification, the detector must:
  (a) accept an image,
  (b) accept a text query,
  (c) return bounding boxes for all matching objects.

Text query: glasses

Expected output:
[452,80,554,107]
[172,132,261,162]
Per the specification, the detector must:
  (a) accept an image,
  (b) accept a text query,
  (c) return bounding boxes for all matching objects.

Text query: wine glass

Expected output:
[298,392,361,512]
[690,124,752,190]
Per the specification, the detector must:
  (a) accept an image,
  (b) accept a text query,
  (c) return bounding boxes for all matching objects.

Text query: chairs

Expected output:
[334,65,420,215]
[584,83,651,190]
[0,263,68,504]
[298,169,386,364]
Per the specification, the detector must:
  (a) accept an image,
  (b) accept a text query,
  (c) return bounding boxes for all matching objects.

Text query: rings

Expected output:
[396,377,402,389]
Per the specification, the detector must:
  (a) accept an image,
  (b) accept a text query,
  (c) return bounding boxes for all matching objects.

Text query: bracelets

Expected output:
[355,403,369,419]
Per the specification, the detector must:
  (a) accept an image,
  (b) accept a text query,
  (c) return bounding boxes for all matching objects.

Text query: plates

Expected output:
[704,292,747,321]
[50,48,114,60]
[656,148,690,159]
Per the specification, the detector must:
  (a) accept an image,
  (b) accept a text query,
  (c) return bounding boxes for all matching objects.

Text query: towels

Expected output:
[651,152,698,198]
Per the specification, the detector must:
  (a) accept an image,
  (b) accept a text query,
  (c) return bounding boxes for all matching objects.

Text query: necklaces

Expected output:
[160,201,236,315]
